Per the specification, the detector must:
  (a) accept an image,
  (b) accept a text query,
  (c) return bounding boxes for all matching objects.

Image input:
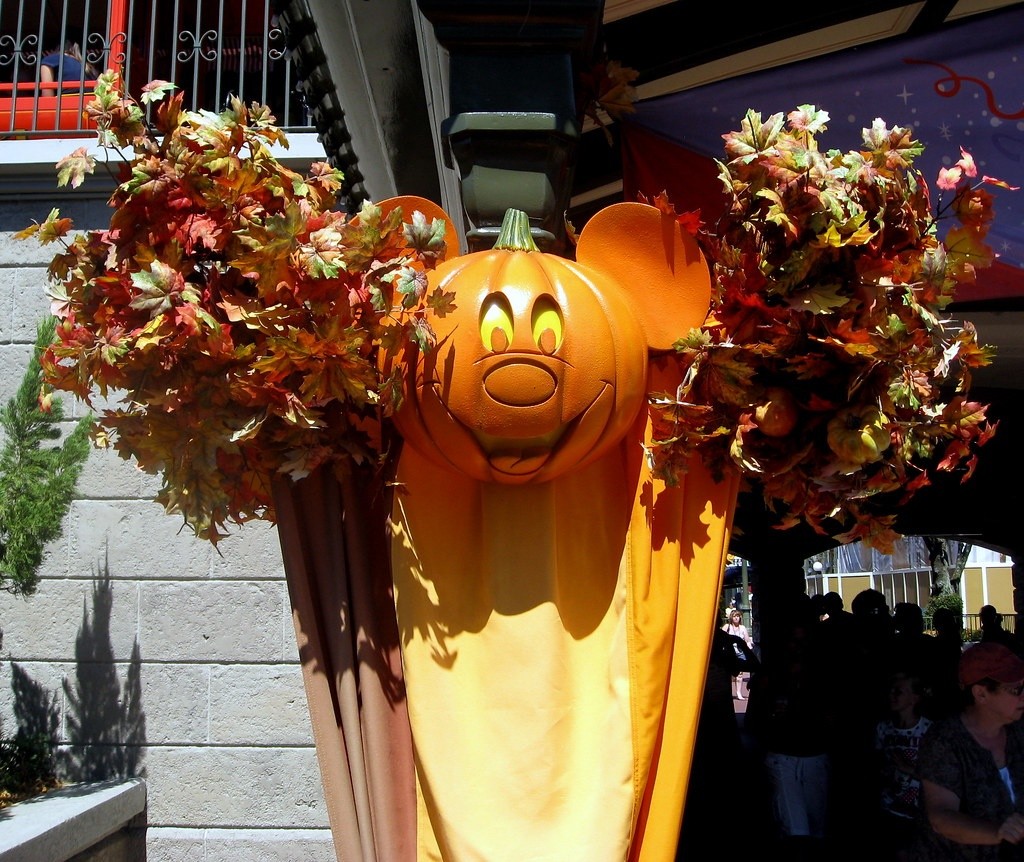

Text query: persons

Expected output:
[906,640,1023,862]
[29,35,99,98]
[678,588,1024,861]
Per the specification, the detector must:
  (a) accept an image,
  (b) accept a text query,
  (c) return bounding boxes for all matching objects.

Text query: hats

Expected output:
[960,642,1024,686]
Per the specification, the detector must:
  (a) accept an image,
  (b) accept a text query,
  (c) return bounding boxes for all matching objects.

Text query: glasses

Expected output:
[991,681,1024,695]
